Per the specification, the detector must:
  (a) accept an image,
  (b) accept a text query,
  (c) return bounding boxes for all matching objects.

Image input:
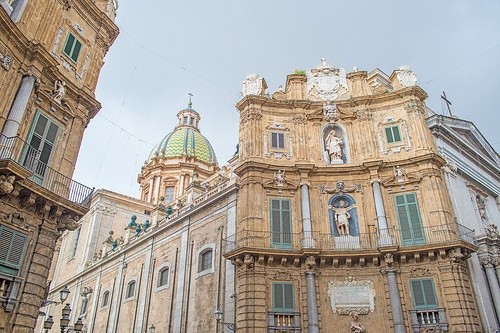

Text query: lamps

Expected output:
[41,285,70,307]
[43,303,84,333]
